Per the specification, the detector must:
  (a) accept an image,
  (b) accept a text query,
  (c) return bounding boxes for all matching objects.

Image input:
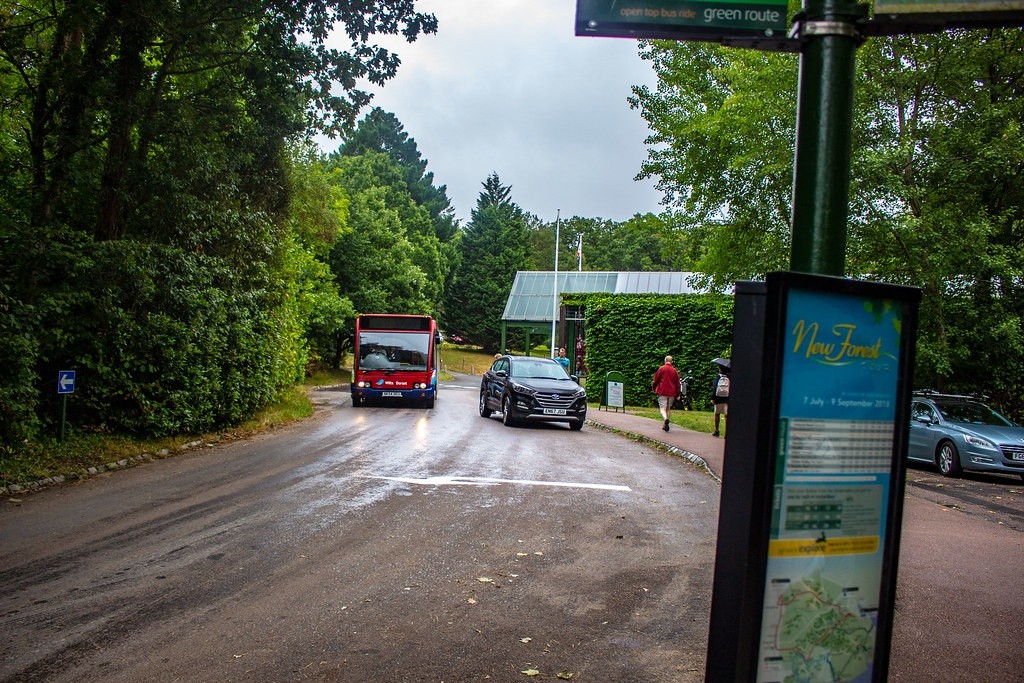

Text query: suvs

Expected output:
[479,355,587,431]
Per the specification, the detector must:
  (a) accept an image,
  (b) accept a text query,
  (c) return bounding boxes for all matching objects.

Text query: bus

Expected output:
[341,312,443,409]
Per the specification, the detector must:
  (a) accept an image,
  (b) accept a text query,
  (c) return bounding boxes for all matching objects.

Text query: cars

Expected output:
[907,392,1024,484]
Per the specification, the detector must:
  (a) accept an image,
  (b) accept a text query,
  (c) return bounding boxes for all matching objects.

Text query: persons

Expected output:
[371,343,386,357]
[651,356,681,432]
[555,348,570,374]
[576,355,590,375]
[494,353,502,360]
[713,368,730,438]
[409,352,424,365]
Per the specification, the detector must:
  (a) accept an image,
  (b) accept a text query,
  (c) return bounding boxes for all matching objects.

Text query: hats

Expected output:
[495,353,502,358]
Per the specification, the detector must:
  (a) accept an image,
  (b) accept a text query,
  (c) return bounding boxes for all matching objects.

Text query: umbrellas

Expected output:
[710,357,732,371]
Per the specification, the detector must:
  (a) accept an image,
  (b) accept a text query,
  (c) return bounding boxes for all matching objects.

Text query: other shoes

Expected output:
[713,430,719,437]
[663,420,670,431]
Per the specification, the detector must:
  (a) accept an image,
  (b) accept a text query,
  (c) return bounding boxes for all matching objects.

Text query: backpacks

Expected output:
[716,374,729,398]
